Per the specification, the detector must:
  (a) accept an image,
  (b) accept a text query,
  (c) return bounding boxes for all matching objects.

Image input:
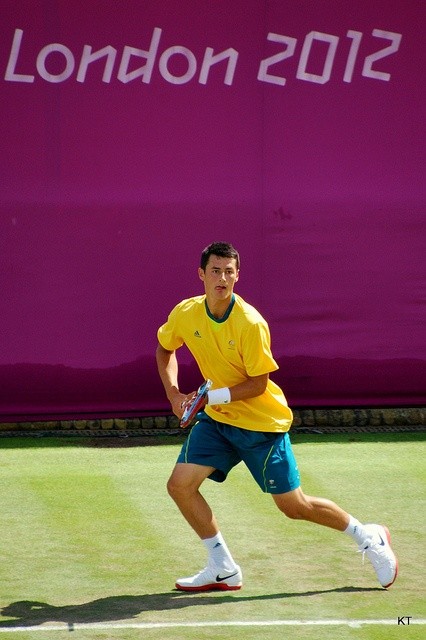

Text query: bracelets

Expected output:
[206,387,231,405]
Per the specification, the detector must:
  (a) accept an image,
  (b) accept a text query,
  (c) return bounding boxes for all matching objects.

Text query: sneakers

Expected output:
[176,568,242,591]
[366,524,396,586]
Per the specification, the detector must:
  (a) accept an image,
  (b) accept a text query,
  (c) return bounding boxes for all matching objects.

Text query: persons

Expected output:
[156,242,398,592]
